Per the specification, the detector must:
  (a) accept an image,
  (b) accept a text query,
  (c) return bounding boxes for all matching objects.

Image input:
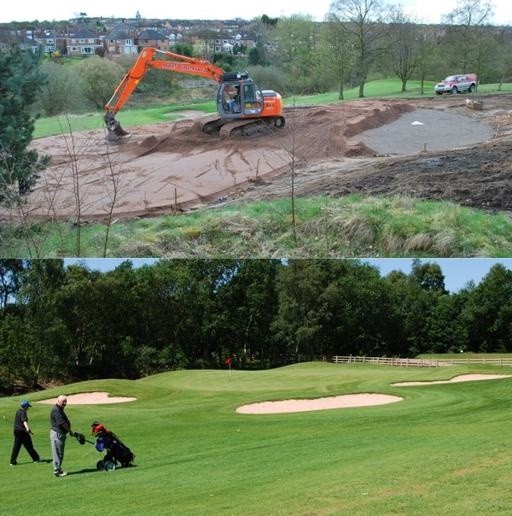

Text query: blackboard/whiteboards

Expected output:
[104,47,286,146]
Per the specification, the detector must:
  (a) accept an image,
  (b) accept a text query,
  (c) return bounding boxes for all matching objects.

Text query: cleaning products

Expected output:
[91,421,135,472]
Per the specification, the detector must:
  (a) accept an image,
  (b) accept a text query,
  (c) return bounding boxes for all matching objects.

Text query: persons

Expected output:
[10,400,43,466]
[228,86,240,112]
[50,395,73,477]
[94,425,120,470]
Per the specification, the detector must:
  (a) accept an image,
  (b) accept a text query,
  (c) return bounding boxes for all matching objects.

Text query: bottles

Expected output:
[20,402,32,407]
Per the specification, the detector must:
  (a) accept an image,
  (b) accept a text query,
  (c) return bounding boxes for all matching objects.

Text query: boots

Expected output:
[434,73,480,95]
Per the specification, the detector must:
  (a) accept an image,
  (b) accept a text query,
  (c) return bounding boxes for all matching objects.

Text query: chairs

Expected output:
[56,472,67,477]
